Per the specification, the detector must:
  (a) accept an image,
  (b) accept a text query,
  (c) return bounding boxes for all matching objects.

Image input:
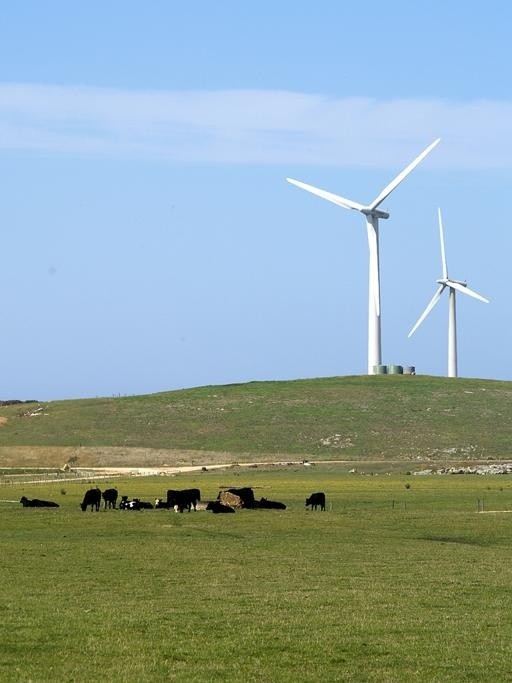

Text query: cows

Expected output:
[79,488,101,512]
[19,496,60,507]
[206,501,236,514]
[119,488,201,513]
[216,487,287,509]
[101,489,118,510]
[305,492,325,511]
